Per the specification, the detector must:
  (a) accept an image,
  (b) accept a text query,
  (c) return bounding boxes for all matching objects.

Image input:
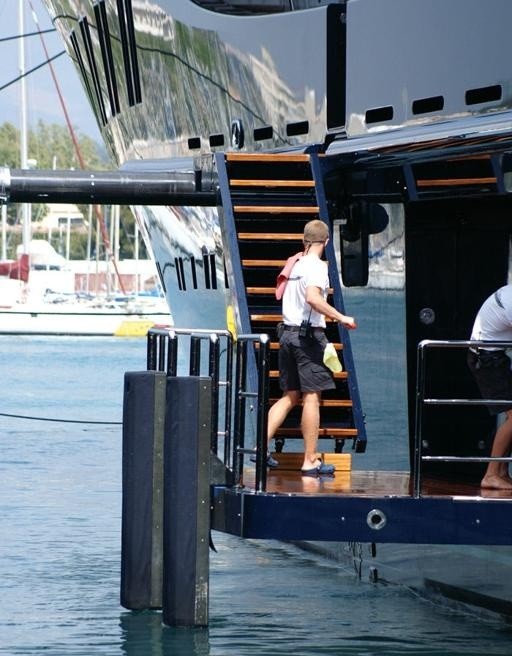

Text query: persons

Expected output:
[251,218,359,475]
[465,279,511,491]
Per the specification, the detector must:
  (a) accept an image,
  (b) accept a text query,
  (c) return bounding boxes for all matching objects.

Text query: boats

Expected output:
[33,0,510,633]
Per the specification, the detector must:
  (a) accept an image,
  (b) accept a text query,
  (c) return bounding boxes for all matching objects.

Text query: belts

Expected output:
[282,324,324,334]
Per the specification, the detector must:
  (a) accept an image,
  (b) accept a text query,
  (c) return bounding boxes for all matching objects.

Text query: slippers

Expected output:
[301,458,335,477]
[251,447,280,468]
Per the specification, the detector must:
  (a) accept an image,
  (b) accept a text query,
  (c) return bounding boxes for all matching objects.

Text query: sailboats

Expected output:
[0,0,179,341]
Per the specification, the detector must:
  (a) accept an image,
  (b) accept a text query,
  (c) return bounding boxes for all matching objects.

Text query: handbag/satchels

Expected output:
[478,352,510,370]
